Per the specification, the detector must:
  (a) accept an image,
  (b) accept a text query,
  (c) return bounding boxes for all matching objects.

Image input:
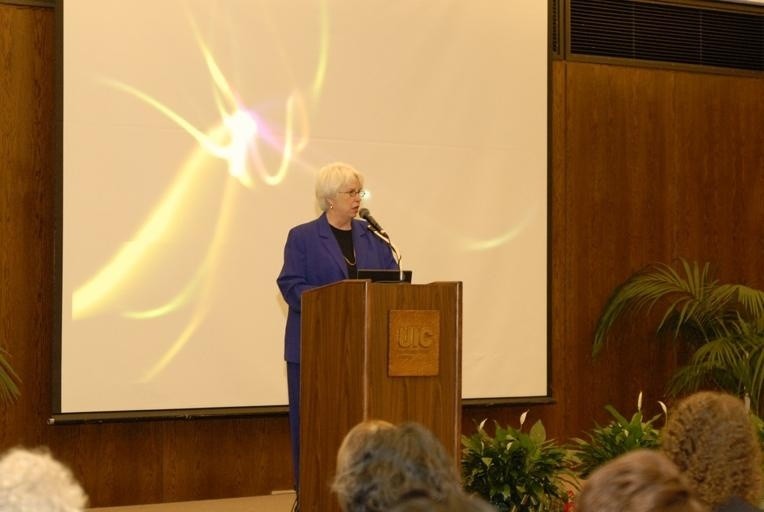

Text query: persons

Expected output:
[574,448,711,510]
[662,389,763,512]
[0,444,89,511]
[275,162,400,506]
[330,420,500,512]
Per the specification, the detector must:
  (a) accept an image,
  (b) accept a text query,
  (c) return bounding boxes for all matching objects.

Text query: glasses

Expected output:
[339,191,365,197]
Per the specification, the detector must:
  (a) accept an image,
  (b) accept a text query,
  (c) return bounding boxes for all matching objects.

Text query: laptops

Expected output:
[356,269,412,284]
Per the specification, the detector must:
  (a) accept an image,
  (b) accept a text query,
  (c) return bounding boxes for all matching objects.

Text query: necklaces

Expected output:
[341,248,356,267]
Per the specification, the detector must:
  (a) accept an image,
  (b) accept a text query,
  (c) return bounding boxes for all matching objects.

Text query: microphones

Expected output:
[359,208,389,239]
